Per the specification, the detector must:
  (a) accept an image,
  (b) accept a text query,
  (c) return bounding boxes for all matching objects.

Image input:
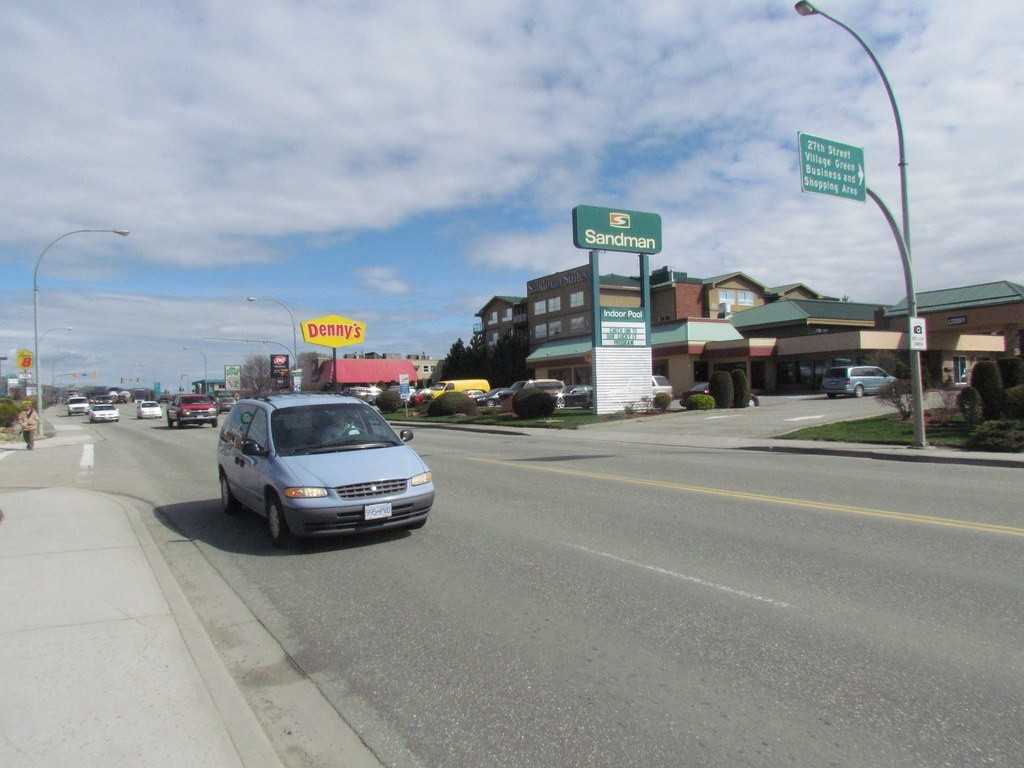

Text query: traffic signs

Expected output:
[796,130,867,203]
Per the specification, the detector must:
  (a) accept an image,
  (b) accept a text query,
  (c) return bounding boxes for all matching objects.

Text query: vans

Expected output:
[429,379,491,399]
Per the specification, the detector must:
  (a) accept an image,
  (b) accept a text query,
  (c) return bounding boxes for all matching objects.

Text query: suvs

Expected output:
[820,366,902,399]
[497,378,567,403]
[166,389,237,428]
[66,397,90,416]
[651,374,674,401]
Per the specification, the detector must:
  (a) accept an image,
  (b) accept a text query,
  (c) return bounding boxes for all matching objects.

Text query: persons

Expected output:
[324,409,360,441]
[19,402,38,448]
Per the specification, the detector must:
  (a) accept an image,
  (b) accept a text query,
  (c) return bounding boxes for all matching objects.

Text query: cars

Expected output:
[472,387,509,408]
[462,390,483,398]
[680,382,760,409]
[64,389,131,405]
[342,385,434,408]
[549,384,594,410]
[88,404,120,424]
[135,401,163,419]
[214,392,434,549]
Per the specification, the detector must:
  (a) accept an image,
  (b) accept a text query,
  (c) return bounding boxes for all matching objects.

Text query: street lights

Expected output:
[181,346,208,395]
[247,296,298,371]
[793,0,926,447]
[50,355,73,405]
[32,229,130,436]
[140,362,157,401]
